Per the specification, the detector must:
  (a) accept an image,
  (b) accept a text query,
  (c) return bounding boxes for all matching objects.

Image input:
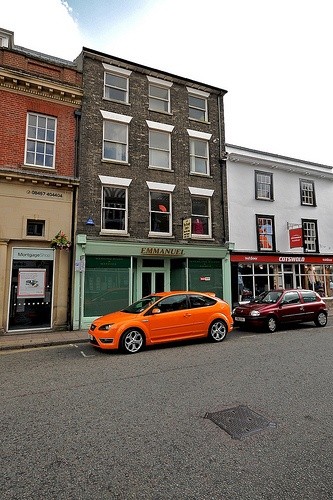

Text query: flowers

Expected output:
[49,230,71,252]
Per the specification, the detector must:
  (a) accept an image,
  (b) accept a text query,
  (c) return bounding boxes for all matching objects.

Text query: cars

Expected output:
[86,290,234,353]
[233,288,327,333]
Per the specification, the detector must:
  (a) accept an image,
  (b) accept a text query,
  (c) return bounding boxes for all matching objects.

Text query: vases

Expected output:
[55,245,70,250]
